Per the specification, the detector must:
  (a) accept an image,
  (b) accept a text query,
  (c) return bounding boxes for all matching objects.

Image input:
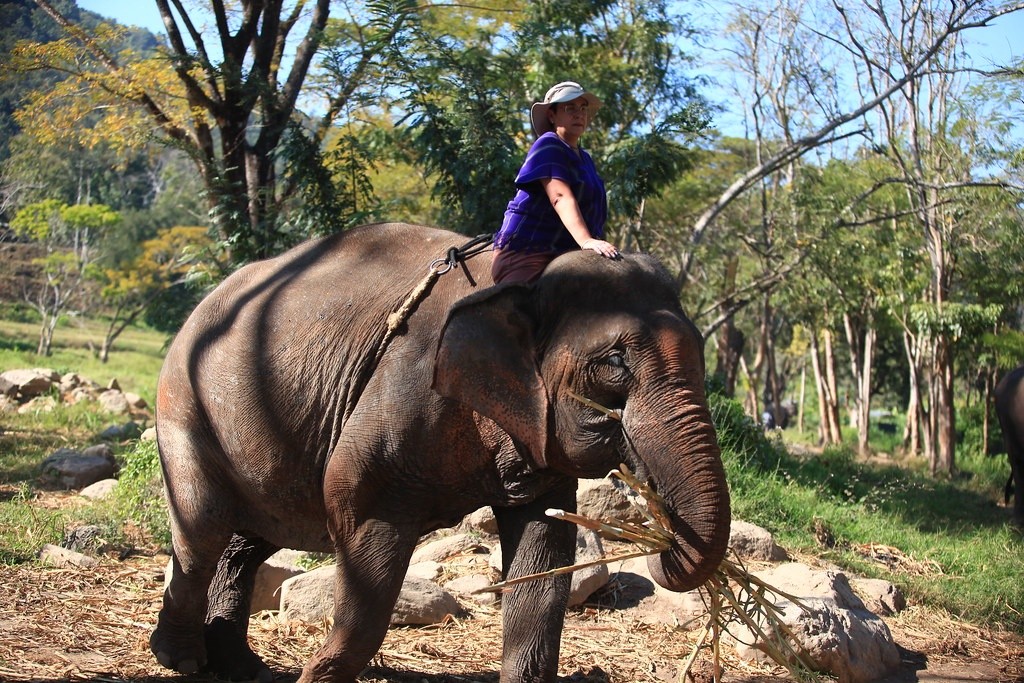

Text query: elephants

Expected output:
[145,220,732,682]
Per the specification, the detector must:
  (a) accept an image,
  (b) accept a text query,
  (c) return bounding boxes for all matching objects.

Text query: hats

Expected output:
[530,81,602,137]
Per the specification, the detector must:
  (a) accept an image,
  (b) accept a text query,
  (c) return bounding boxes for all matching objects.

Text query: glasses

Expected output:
[558,104,589,114]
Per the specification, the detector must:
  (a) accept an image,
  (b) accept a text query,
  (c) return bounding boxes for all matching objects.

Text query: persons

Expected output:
[763,403,798,430]
[491,81,619,282]
